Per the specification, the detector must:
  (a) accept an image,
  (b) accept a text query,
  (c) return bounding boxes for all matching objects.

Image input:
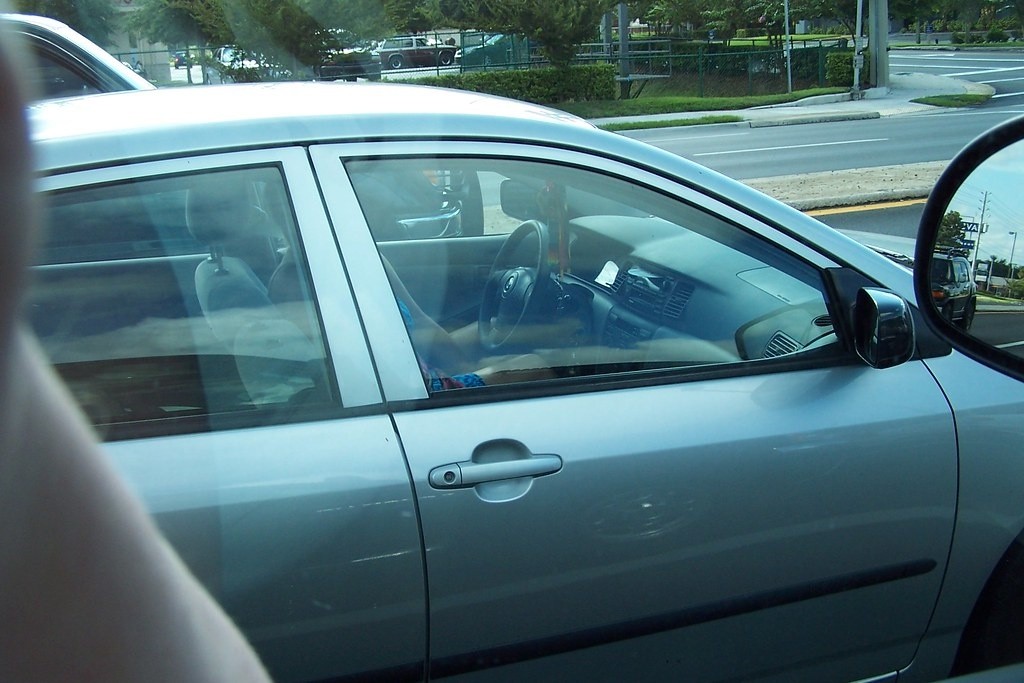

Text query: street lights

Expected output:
[1006,231,1017,281]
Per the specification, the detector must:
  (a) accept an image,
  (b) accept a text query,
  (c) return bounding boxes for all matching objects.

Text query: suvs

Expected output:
[174,52,194,69]
[374,37,459,71]
[928,243,978,332]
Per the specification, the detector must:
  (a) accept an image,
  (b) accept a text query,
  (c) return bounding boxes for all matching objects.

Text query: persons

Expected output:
[262,167,585,396]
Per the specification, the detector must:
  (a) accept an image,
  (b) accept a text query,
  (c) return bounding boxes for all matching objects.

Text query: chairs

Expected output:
[185,177,320,410]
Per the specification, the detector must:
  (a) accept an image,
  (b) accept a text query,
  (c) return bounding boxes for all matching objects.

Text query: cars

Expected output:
[453,33,519,70]
[25,79,1023,682]
[314,29,381,64]
[213,45,262,68]
[0,13,154,105]
